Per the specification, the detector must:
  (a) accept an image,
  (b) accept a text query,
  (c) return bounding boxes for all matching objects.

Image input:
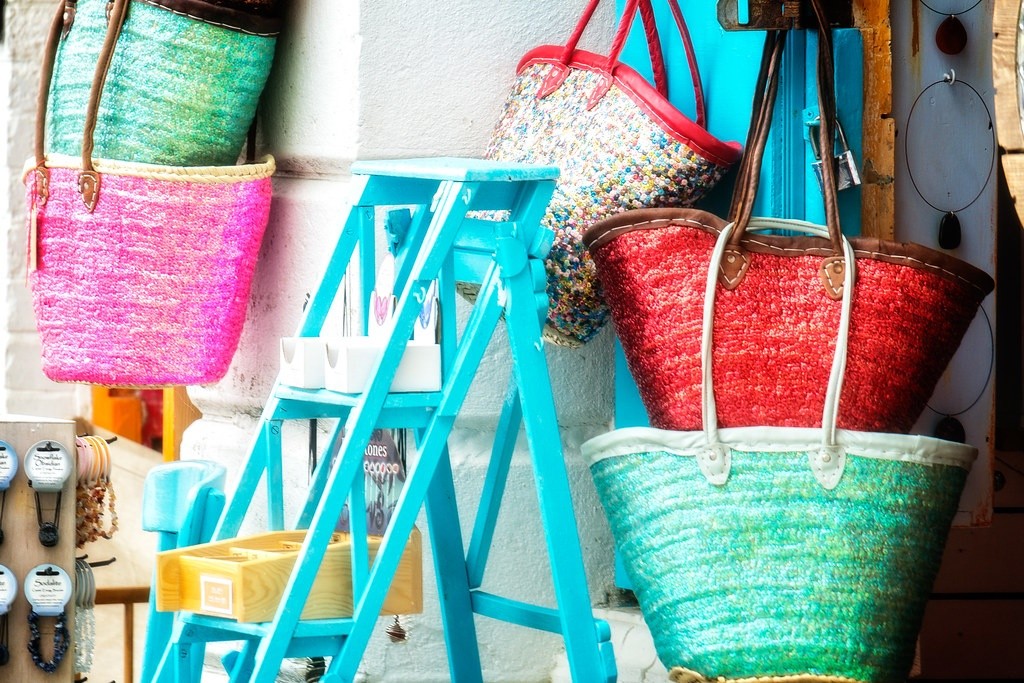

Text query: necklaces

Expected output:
[75,480,119,550]
[926,303,994,445]
[73,606,96,674]
[0,489,6,545]
[905,80,995,249]
[0,611,8,666]
[26,609,71,673]
[34,490,61,547]
[920,0,984,55]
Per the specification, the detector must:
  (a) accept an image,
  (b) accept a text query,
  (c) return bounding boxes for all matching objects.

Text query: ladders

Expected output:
[154,156,619,683]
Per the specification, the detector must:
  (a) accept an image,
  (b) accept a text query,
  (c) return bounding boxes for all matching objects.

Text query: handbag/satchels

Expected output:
[451,0,742,349]
[23,0,293,389]
[585,0,996,682]
